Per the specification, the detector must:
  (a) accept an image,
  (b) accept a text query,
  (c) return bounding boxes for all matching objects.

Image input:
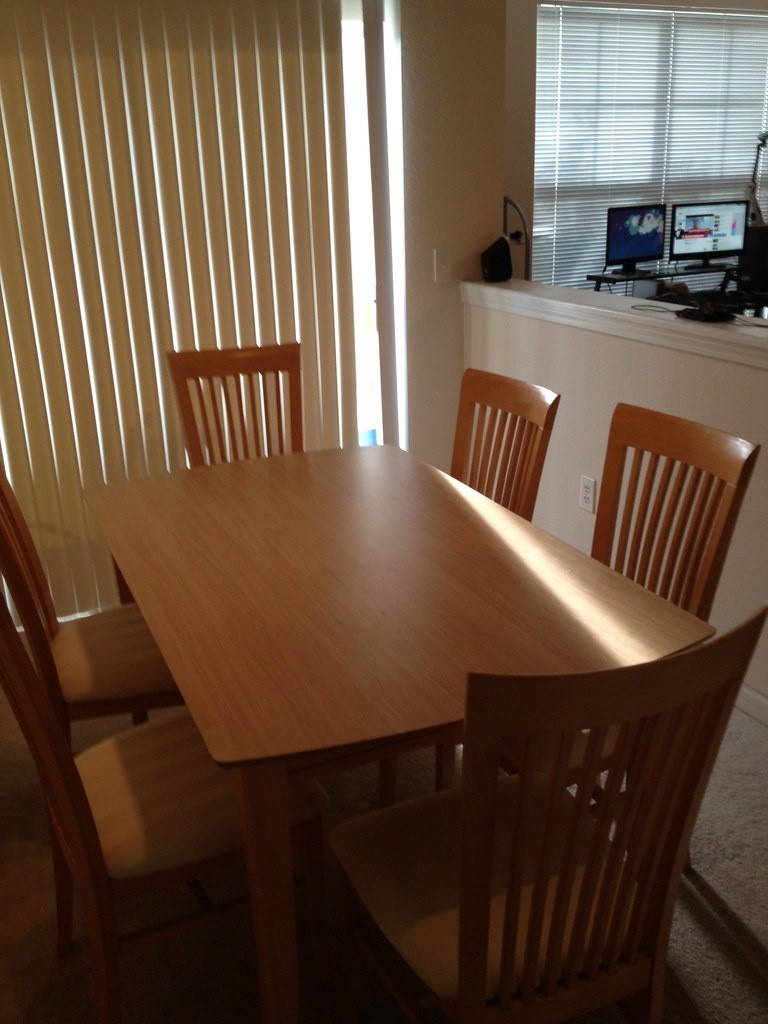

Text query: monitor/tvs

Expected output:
[669,200,750,271]
[605,204,666,276]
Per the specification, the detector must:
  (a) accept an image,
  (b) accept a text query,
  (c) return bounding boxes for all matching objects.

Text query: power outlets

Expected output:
[579,475,595,515]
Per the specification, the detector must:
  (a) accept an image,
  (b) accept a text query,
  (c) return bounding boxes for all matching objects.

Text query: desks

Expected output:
[586,262,738,292]
[81,441,715,1024]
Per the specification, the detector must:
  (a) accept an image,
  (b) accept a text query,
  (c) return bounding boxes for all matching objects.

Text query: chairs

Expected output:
[0,587,333,1023]
[375,368,560,810]
[165,341,303,466]
[434,404,761,805]
[331,604,768,1024]
[0,447,187,746]
